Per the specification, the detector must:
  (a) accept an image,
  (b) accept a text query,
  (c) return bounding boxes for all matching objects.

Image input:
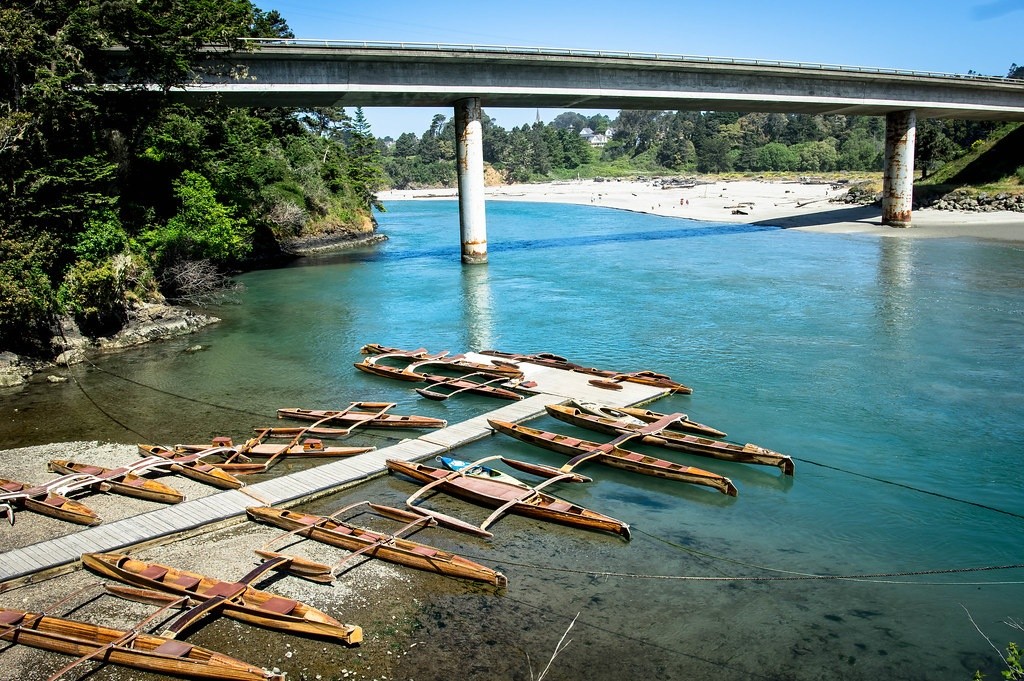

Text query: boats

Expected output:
[1,337,794,681]
